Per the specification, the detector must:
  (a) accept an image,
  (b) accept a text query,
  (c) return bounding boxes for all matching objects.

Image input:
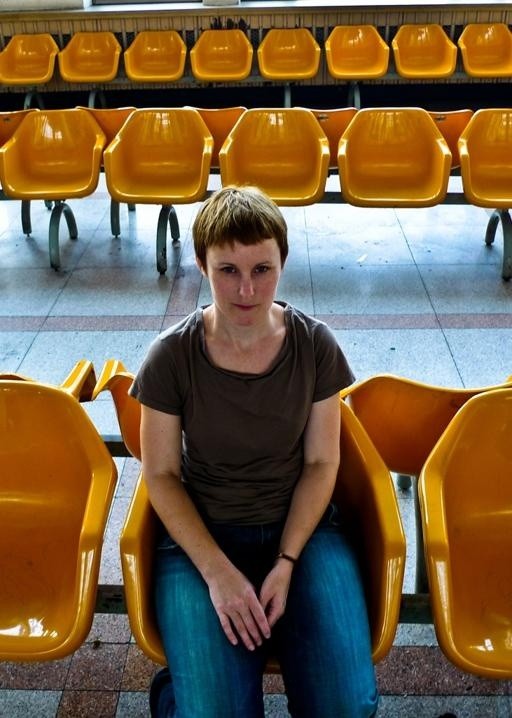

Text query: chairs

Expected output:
[0,379,119,662]
[349,374,512,477]
[119,398,406,673]
[417,388,512,680]
[92,358,352,462]
[0,358,97,403]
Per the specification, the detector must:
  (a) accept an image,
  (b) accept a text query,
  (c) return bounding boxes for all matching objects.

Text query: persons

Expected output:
[128,184,381,718]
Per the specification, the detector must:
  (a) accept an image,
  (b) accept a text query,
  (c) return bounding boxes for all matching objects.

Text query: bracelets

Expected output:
[274,553,296,565]
